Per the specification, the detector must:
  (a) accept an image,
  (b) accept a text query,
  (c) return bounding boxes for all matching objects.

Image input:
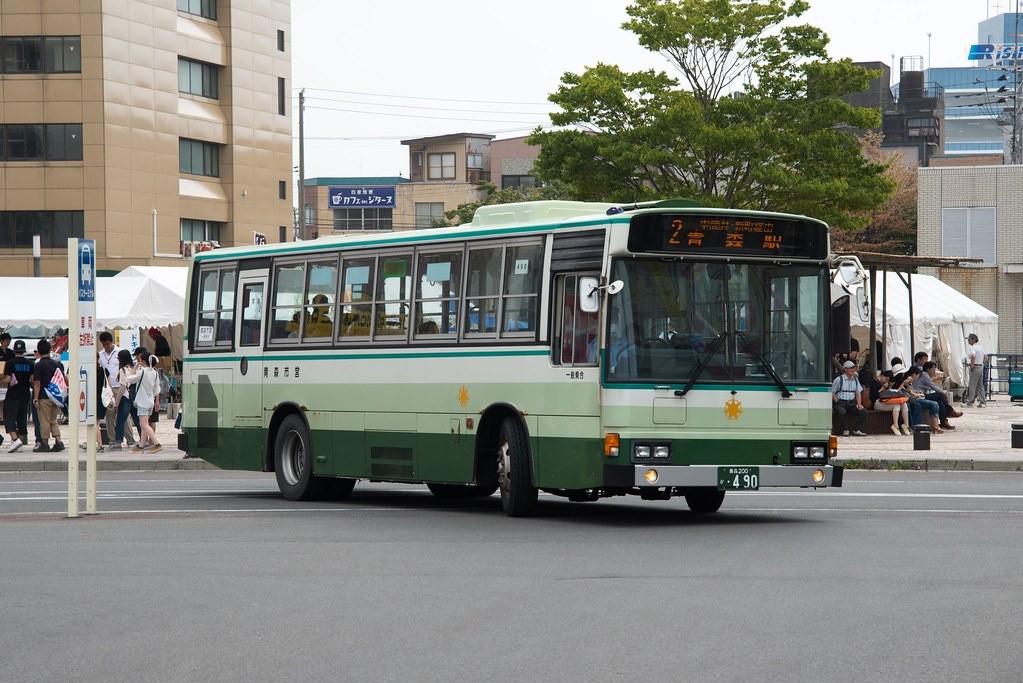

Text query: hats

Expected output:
[14,340,26,349]
[842,361,858,369]
[892,363,908,378]
[965,333,978,339]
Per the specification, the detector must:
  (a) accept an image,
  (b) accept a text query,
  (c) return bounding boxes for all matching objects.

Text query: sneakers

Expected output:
[901,424,913,435]
[891,425,901,436]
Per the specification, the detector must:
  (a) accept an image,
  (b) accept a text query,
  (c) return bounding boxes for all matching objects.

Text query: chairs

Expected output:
[671,333,710,371]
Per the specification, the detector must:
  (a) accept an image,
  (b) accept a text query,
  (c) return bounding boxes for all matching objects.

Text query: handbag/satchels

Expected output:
[42,367,67,407]
[101,365,116,412]
[879,388,909,404]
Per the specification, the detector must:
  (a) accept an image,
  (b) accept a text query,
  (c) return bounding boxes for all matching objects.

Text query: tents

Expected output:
[1,258,529,330]
[735,268,999,391]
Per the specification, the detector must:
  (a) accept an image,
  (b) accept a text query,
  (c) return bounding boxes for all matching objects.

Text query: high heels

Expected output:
[932,424,944,434]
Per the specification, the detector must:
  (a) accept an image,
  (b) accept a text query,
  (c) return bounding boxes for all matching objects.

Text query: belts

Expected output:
[974,364,982,366]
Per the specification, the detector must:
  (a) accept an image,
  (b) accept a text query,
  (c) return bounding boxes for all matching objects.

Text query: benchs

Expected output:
[831,409,932,434]
[202,318,287,343]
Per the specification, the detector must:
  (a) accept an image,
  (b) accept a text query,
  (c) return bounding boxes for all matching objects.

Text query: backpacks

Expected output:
[129,370,158,400]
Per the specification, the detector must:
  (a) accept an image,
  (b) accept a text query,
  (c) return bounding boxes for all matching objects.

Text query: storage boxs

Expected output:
[155,355,172,371]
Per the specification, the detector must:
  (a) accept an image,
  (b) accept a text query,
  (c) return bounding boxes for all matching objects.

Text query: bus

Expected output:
[178,200,871,517]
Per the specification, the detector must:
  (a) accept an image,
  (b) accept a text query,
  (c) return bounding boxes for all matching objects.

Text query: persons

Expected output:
[284,294,441,337]
[778,333,964,435]
[960,333,988,407]
[0,325,202,459]
[587,306,679,378]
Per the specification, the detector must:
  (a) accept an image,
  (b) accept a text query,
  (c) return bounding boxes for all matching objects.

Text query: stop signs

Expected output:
[79,391,86,411]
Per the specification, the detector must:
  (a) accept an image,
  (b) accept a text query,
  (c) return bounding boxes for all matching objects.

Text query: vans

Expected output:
[3,321,62,358]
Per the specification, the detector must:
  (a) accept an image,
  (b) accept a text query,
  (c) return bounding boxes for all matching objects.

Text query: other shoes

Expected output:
[17,435,27,445]
[843,430,850,437]
[947,411,964,418]
[52,441,65,452]
[7,438,23,453]
[79,430,164,454]
[35,441,42,448]
[961,403,973,408]
[940,420,956,430]
[851,430,866,436]
[977,404,985,408]
[33,443,51,453]
